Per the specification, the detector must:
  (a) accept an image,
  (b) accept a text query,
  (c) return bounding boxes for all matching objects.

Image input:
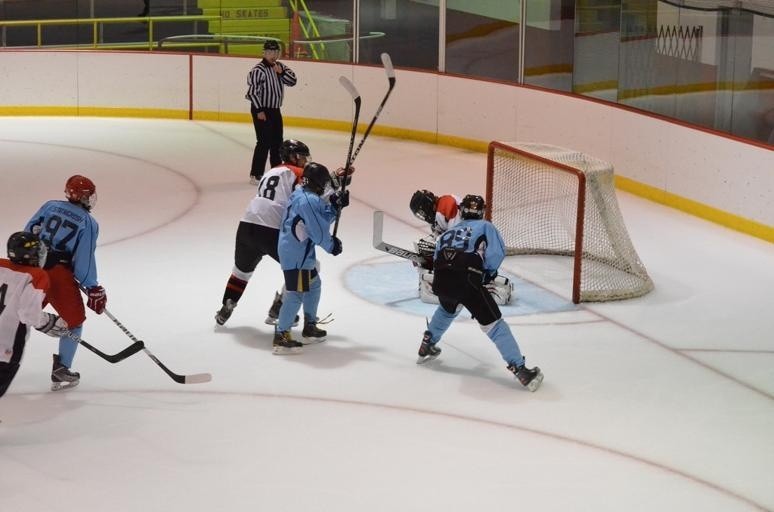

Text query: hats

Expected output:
[264,39,280,50]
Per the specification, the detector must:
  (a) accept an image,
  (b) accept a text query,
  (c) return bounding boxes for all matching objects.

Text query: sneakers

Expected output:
[273,324,303,348]
[302,312,334,338]
[215,298,237,325]
[251,175,262,181]
[418,331,441,357]
[268,290,300,322]
[506,356,540,386]
[51,353,80,382]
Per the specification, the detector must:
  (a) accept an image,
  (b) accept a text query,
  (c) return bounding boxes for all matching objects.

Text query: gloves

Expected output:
[330,190,349,211]
[335,166,355,177]
[35,312,68,337]
[87,285,107,314]
[332,236,342,256]
[483,269,498,285]
[331,176,351,190]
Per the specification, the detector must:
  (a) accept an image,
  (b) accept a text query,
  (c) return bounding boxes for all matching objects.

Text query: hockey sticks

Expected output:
[374,210,508,285]
[334,76,361,237]
[74,279,212,384]
[346,53,395,165]
[64,330,145,363]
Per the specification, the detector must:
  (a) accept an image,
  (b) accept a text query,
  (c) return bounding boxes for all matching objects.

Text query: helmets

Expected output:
[279,139,312,163]
[458,194,485,220]
[300,162,331,195]
[409,189,438,224]
[64,175,98,210]
[7,231,48,269]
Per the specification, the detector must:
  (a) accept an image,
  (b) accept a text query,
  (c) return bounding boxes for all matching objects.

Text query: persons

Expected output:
[215,139,354,326]
[273,161,350,347]
[242,39,297,186]
[417,195,540,386]
[22,175,106,383]
[409,190,514,305]
[0,229,69,399]
